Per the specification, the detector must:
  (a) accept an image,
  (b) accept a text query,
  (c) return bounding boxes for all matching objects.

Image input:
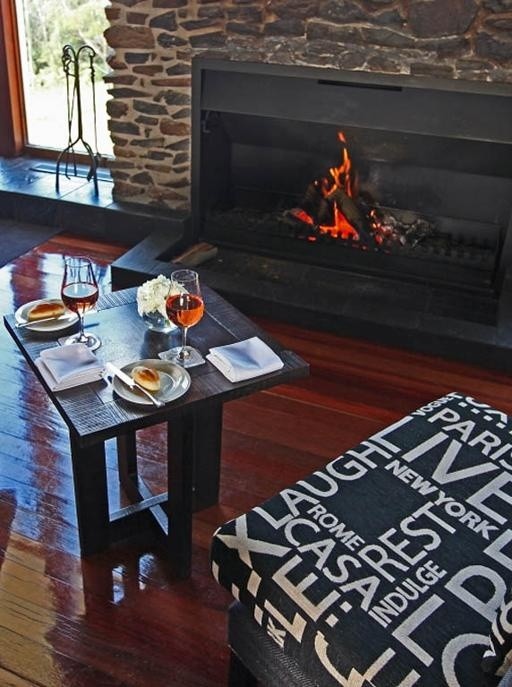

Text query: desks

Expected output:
[4,281,312,585]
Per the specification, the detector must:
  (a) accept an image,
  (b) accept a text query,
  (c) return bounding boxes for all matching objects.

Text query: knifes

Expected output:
[14,310,97,328]
[105,358,165,409]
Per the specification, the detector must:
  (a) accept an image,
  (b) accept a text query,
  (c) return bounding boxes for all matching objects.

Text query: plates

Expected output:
[111,357,193,407]
[11,298,78,332]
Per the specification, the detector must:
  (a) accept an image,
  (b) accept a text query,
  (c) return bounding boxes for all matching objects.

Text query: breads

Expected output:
[27,302,65,322]
[135,368,161,391]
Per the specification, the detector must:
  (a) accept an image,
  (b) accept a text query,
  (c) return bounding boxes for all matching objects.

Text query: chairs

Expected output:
[210,390,512,686]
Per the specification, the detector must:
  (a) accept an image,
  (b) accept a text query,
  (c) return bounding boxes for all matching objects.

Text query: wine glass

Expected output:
[61,257,102,352]
[166,269,204,364]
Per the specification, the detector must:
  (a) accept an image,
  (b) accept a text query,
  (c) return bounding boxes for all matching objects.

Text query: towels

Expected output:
[204,336,287,384]
[32,343,106,393]
